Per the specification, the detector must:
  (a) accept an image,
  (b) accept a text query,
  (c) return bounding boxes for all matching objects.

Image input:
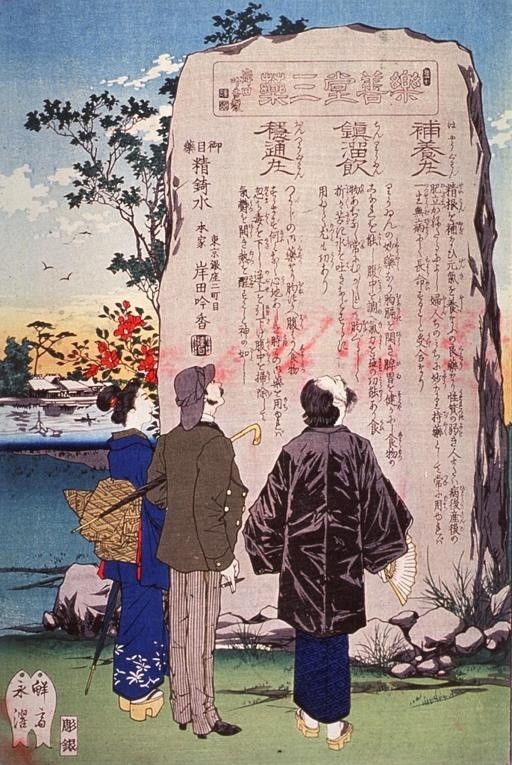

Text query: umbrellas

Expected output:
[85,581,120,696]
[72,422,260,533]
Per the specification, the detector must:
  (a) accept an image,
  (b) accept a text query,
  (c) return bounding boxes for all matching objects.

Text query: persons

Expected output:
[146,364,248,741]
[243,375,413,751]
[95,379,170,720]
